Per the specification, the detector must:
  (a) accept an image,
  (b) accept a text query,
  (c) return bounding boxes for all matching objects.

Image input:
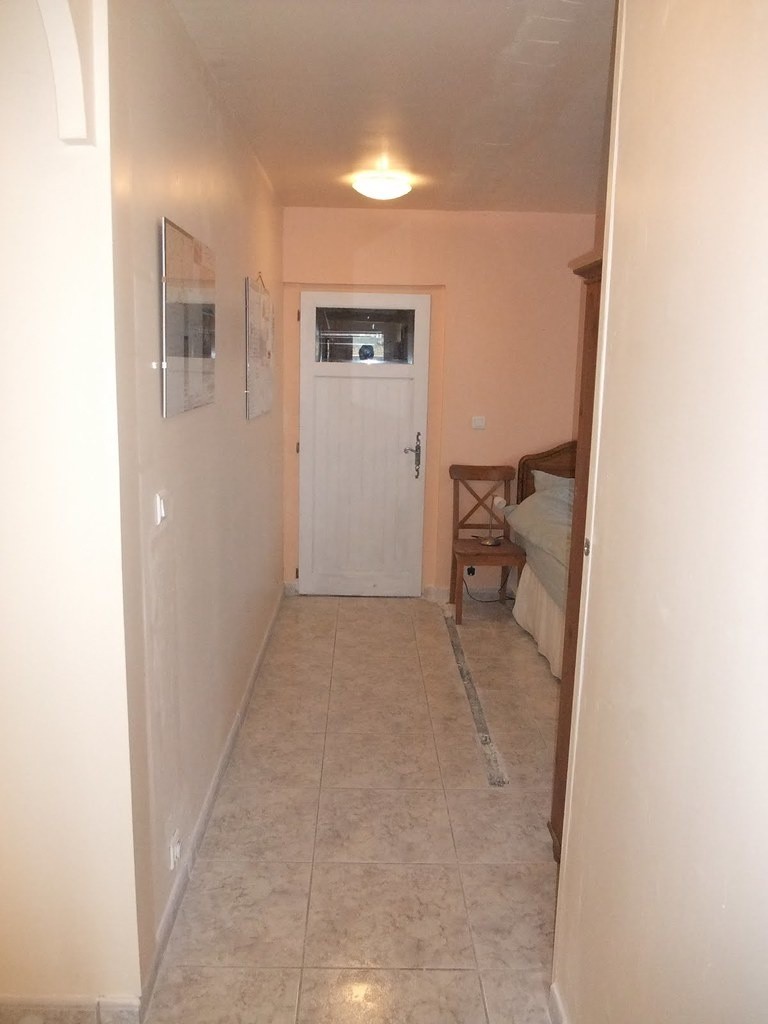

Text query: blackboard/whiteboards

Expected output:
[243,276,276,422]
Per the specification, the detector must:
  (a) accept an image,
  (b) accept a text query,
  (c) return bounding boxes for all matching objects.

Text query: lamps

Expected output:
[352,173,412,200]
[480,495,507,546]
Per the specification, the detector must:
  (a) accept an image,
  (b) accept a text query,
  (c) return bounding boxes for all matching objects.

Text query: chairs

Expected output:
[449,465,526,625]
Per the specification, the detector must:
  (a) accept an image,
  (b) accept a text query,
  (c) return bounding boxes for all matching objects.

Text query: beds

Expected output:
[512,440,579,681]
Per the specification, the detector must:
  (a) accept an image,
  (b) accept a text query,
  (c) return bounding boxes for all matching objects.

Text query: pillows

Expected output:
[531,470,575,492]
